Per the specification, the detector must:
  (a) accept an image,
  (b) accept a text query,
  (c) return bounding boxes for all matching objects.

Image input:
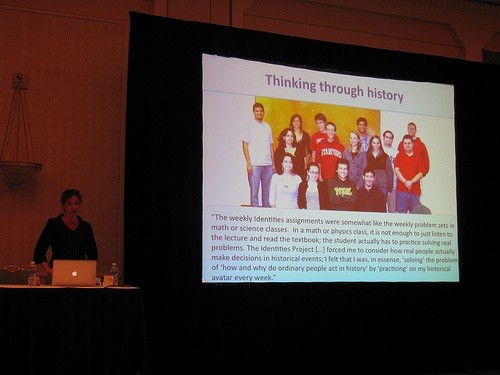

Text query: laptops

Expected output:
[51,260,97,286]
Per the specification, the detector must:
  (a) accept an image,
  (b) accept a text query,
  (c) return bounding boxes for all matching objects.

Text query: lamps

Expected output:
[0,71,43,187]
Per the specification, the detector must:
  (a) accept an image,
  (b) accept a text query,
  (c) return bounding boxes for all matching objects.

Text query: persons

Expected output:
[241,103,275,207]
[268,154,302,210]
[33,190,99,284]
[316,122,345,182]
[395,135,423,213]
[289,114,310,166]
[368,136,393,203]
[342,131,367,190]
[274,128,305,180]
[358,167,387,212]
[298,162,329,210]
[356,118,372,152]
[381,131,399,212]
[398,123,430,180]
[327,160,360,211]
[310,113,339,162]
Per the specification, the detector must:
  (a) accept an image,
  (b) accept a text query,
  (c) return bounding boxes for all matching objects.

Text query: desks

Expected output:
[0,284,150,375]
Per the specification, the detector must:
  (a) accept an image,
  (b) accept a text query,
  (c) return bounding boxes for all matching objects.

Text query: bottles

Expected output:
[28,262,37,277]
[110,263,118,286]
